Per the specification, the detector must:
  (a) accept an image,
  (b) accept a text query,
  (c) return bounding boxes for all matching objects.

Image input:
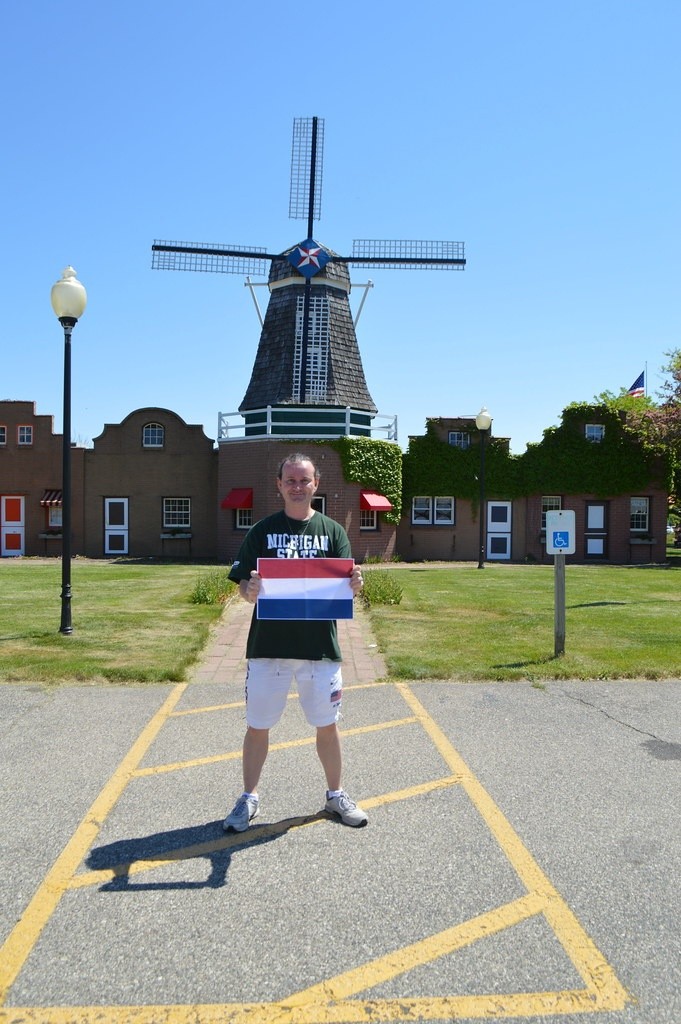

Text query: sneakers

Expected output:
[222,795,259,832]
[324,791,368,827]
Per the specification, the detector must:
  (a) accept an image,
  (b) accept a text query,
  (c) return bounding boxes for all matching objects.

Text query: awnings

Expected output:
[39,490,62,506]
[221,488,252,509]
[360,491,392,511]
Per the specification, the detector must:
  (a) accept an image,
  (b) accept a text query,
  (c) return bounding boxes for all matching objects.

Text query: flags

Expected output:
[627,370,644,398]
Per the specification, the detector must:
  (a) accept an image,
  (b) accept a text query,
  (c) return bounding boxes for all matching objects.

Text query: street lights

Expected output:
[50,266,86,630]
[477,408,493,569]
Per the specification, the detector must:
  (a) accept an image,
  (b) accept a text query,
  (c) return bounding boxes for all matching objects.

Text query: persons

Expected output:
[222,454,368,832]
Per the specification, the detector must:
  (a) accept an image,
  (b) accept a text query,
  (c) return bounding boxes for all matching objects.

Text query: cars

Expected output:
[667,526,674,533]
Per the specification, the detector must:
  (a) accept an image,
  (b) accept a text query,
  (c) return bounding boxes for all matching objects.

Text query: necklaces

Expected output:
[287,514,311,550]
